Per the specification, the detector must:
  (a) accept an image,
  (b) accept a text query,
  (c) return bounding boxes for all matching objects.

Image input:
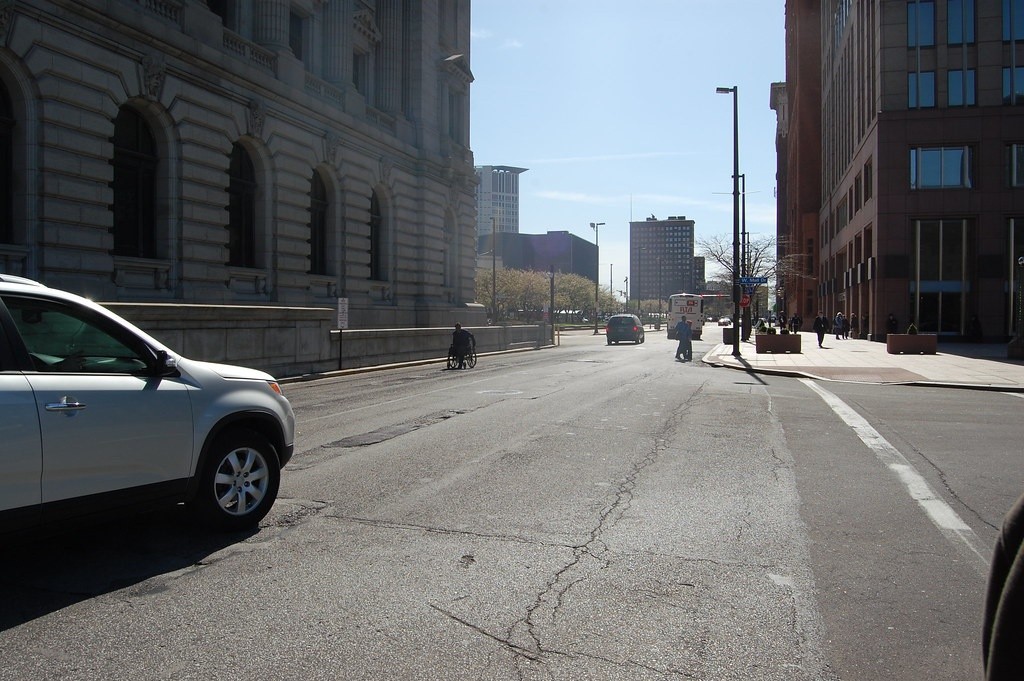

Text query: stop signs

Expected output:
[740,295,749,307]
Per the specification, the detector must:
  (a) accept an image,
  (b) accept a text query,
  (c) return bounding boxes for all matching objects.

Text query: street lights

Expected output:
[731,173,747,342]
[716,86,739,355]
[489,215,497,325]
[595,222,605,333]
[637,247,647,320]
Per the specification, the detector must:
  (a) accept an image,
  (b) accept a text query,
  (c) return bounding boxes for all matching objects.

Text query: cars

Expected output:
[0,273,295,538]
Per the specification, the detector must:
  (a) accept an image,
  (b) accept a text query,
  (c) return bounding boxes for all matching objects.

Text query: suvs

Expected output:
[605,313,644,345]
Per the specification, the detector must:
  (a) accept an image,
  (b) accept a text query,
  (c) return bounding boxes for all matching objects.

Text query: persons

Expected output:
[841,315,850,339]
[886,314,897,333]
[675,316,693,360]
[813,311,829,349]
[851,313,858,329]
[778,311,787,329]
[789,313,802,334]
[861,315,868,332]
[453,323,476,369]
[31,351,87,373]
[834,312,842,340]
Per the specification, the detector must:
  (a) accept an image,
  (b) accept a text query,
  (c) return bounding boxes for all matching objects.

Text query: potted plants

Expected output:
[755,319,801,353]
[887,324,937,354]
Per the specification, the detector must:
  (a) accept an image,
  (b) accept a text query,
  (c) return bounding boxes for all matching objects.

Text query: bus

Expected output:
[667,292,703,340]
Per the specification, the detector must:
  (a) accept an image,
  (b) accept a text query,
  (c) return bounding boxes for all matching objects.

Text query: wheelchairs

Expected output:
[447,336,477,370]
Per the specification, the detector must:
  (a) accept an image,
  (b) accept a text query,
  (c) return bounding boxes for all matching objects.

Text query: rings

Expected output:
[80,366,82,369]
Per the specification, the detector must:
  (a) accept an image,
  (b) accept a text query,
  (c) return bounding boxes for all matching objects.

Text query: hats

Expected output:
[687,320,692,323]
